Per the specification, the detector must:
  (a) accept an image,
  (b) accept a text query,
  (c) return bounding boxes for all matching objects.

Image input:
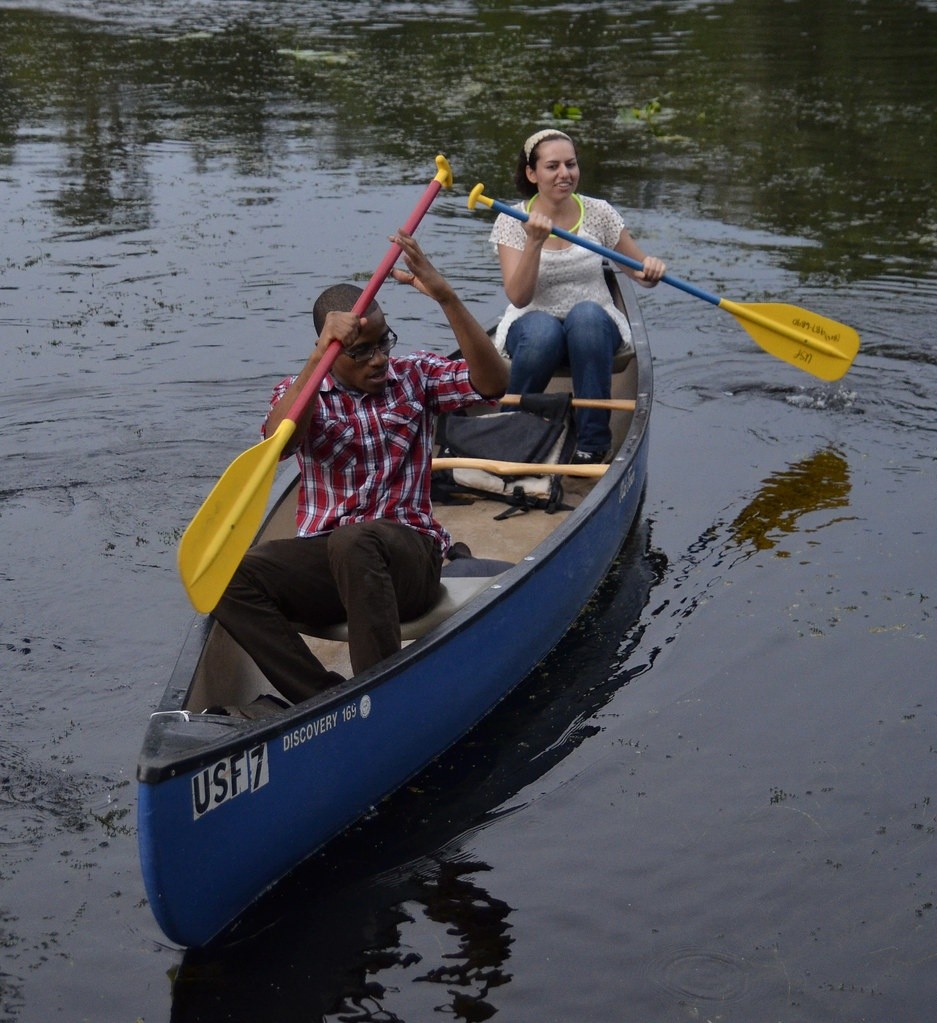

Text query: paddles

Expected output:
[467,183,862,383]
[177,153,454,616]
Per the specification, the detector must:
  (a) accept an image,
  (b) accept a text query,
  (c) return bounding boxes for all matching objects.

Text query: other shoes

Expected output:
[568,446,613,479]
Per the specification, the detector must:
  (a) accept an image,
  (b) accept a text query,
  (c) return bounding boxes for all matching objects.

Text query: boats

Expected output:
[135,262,654,954]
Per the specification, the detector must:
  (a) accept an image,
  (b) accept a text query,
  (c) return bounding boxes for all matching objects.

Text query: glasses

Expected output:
[342,323,399,362]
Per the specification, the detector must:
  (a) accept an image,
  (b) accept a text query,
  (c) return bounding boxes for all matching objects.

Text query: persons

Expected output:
[209,226,512,706]
[489,129,667,478]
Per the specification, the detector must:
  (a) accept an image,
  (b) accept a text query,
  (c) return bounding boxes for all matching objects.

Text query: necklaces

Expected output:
[527,192,585,238]
